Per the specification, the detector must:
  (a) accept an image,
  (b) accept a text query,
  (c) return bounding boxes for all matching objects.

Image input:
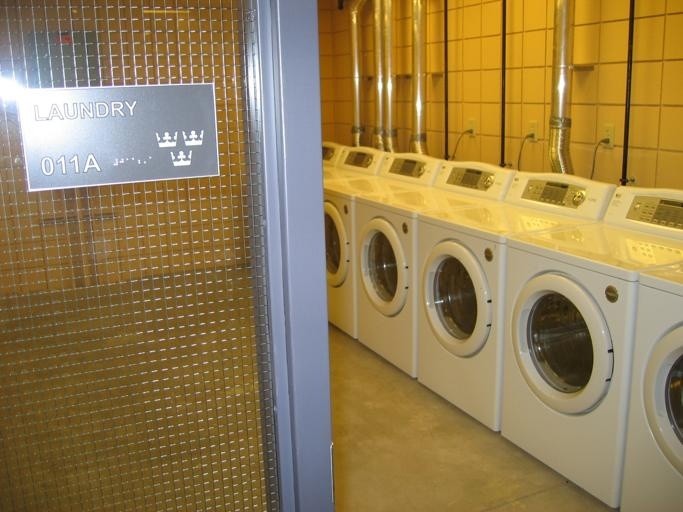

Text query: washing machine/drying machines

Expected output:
[322,139,683,512]
